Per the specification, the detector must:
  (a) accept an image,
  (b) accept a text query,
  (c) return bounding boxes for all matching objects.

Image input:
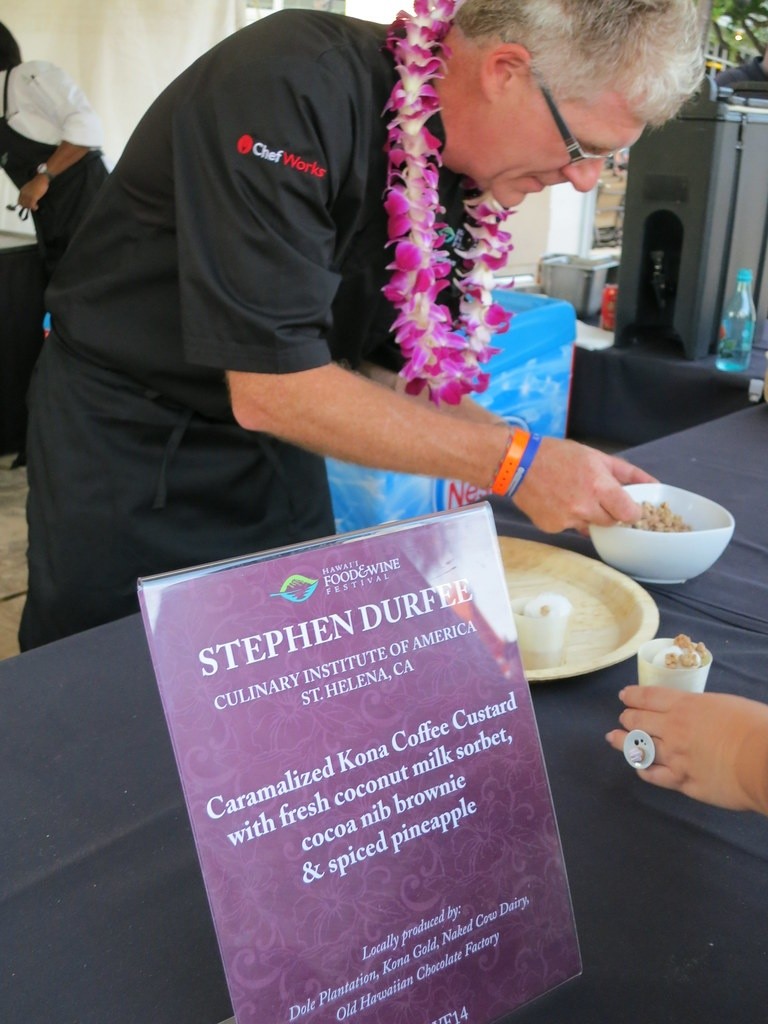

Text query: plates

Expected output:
[495,534,659,683]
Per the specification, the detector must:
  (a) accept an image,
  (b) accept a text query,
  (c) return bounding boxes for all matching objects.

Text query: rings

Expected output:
[623,729,657,771]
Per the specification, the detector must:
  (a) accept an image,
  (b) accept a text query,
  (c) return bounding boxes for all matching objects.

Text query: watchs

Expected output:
[37,163,54,181]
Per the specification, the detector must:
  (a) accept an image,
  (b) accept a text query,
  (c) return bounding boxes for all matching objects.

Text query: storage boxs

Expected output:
[540,254,619,316]
[323,290,581,533]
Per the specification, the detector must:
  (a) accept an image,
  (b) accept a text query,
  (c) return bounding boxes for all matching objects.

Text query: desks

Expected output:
[565,317,768,449]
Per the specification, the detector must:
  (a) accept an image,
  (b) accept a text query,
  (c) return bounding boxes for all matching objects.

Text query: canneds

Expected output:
[601,284,616,331]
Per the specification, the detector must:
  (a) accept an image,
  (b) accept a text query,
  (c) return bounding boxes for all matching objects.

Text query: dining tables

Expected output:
[0,398,768,1024]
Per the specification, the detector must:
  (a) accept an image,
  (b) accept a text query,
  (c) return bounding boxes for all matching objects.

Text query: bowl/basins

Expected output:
[588,479,736,585]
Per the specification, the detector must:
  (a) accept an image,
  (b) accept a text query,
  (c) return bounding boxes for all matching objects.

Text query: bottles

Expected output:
[599,282,619,333]
[714,268,756,372]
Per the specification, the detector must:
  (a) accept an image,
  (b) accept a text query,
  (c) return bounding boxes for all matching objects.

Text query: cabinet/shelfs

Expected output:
[0,229,48,459]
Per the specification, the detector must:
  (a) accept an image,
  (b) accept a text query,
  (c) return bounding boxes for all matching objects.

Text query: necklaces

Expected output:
[380,0,515,407]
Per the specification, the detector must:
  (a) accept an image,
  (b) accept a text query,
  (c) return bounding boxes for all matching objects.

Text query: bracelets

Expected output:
[489,427,542,500]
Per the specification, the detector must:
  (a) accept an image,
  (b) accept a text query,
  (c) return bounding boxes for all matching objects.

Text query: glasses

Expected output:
[538,85,627,163]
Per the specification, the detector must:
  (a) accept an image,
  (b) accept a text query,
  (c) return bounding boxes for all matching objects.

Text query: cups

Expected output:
[637,635,712,698]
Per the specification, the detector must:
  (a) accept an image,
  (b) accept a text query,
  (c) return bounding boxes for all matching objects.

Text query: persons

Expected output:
[603,685,768,817]
[16,2,711,663]
[0,60,118,469]
[713,41,768,98]
